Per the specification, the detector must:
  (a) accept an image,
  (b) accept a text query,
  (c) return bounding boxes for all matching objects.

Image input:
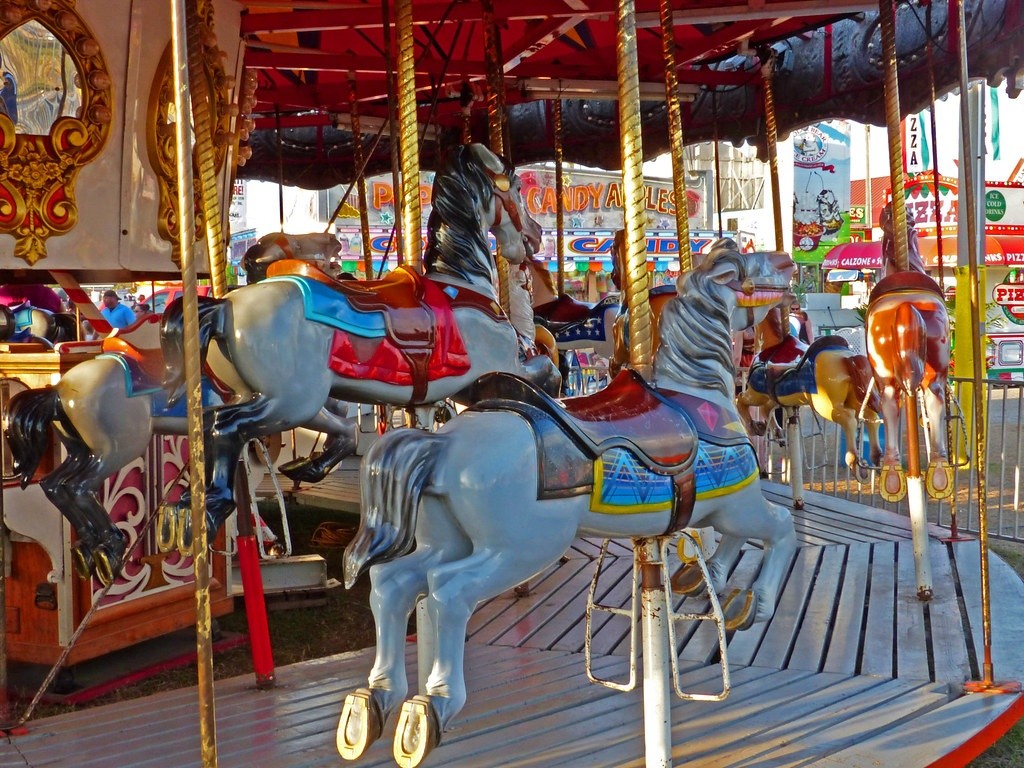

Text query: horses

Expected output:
[508,229,677,380]
[336,238,798,767]
[3,143,542,587]
[737,307,884,484]
[865,202,954,503]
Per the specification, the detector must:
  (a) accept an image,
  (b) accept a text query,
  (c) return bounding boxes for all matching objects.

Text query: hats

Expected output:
[104,290,121,299]
[58,290,67,297]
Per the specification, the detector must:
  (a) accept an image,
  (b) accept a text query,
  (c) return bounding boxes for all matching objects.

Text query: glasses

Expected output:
[134,310,143,313]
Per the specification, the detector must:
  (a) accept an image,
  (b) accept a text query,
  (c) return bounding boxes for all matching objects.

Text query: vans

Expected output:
[132,285,248,318]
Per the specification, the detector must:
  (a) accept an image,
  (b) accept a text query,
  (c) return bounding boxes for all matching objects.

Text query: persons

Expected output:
[134,304,150,319]
[99,290,136,328]
